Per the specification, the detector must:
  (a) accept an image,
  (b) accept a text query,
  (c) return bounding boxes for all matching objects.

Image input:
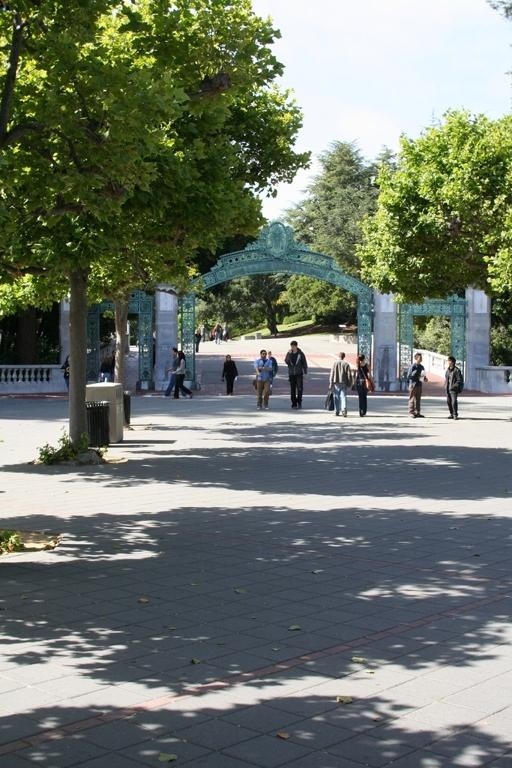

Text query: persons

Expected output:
[254,349,272,410]
[162,348,187,399]
[285,341,307,409]
[406,353,428,418]
[350,354,372,418]
[60,354,69,391]
[445,356,463,420]
[194,322,229,353]
[222,354,238,396]
[172,351,192,399]
[329,352,352,417]
[100,350,115,382]
[266,351,278,395]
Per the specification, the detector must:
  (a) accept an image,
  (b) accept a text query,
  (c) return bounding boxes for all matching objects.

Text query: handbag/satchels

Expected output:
[253,380,257,390]
[324,390,334,411]
[365,378,374,394]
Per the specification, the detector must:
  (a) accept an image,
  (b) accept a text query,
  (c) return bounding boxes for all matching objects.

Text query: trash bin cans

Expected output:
[83,401,111,447]
[122,391,130,425]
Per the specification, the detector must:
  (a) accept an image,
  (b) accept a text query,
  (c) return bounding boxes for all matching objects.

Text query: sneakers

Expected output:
[414,413,424,417]
[452,414,457,420]
[448,415,454,419]
